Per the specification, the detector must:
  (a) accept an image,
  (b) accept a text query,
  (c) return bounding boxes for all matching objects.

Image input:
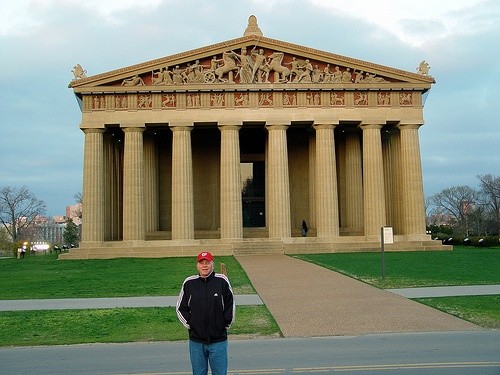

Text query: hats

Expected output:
[197,252,213,262]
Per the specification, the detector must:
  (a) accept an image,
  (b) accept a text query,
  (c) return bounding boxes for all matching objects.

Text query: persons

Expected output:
[176,252,234,375]
[301,220,307,237]
[118,48,389,85]
